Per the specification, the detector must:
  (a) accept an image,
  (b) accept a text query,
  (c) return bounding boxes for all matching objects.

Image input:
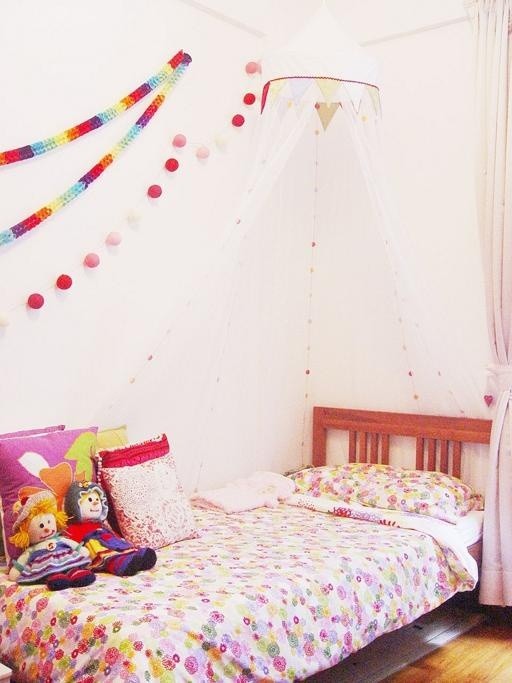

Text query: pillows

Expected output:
[288,462,485,525]
[0,424,202,571]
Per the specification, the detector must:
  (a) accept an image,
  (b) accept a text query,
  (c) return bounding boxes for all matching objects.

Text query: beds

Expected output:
[0,406,492,682]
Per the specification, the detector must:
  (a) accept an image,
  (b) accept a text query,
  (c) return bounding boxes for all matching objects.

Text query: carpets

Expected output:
[297,602,490,682]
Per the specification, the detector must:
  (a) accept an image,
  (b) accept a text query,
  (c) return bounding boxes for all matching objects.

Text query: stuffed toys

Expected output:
[8,491,96,591]
[62,480,157,578]
